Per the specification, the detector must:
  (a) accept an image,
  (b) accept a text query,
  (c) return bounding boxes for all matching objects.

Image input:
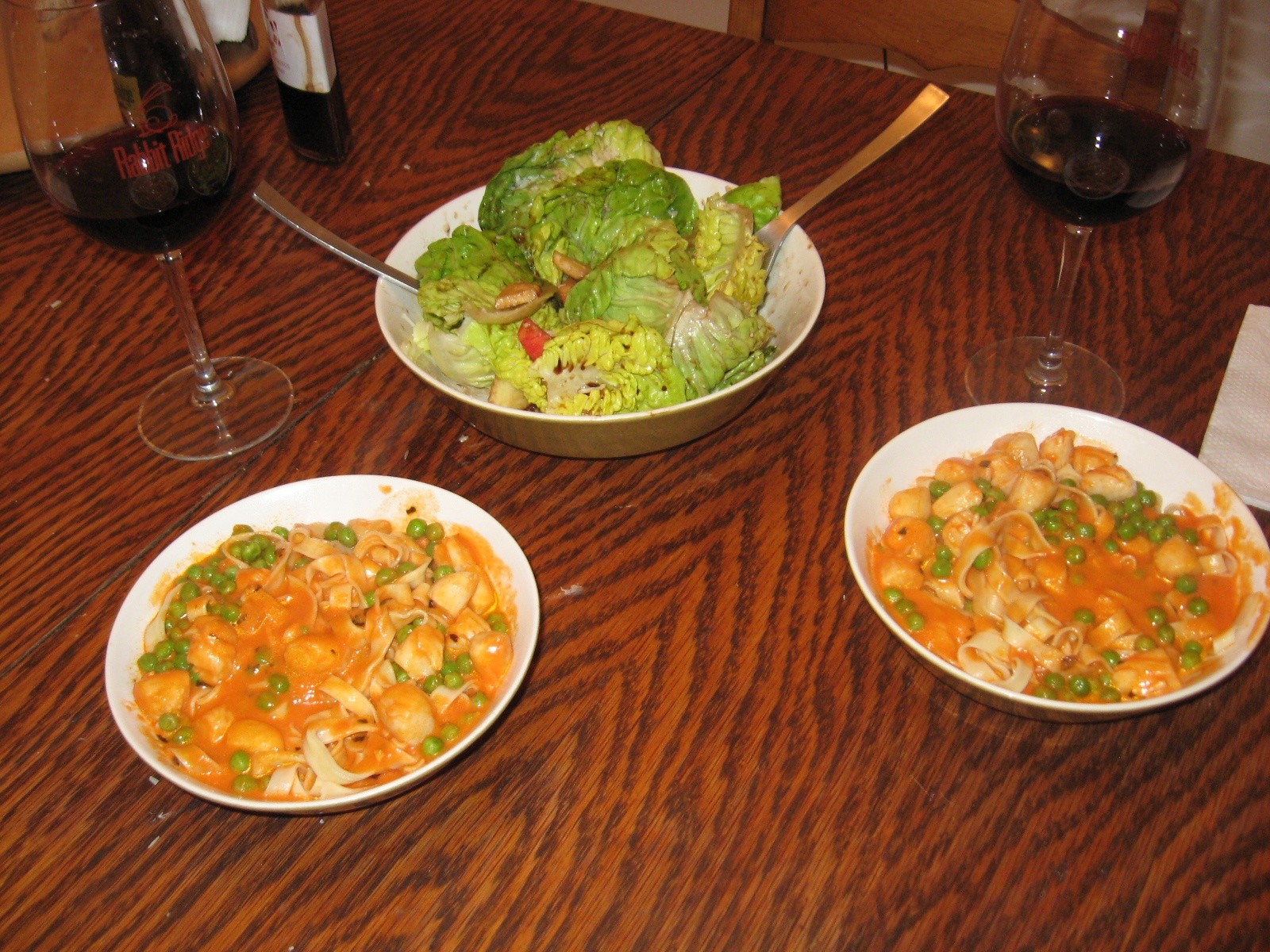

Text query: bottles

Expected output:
[261,1,350,167]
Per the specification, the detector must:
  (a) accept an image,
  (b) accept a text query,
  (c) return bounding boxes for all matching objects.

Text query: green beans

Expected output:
[881,475,1210,701]
[137,521,510,797]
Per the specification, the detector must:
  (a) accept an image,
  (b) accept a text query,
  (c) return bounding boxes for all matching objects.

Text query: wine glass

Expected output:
[963,1,1231,419]
[7,0,294,461]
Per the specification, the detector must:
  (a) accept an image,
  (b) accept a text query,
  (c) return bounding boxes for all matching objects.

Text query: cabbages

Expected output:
[402,118,781,417]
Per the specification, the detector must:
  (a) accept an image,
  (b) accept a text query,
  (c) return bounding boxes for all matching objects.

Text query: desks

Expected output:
[0,1,1268,952]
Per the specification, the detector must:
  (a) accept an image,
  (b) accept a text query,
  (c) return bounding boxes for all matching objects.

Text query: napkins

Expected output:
[1200,305,1270,512]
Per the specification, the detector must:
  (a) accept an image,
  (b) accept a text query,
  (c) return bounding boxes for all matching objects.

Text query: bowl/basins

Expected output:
[375,167,826,459]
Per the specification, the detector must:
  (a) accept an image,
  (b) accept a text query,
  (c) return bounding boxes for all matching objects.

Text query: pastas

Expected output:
[133,521,503,800]
[911,453,1240,700]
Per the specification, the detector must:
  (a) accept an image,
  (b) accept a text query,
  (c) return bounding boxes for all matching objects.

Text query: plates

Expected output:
[105,473,542,815]
[843,401,1270,725]
